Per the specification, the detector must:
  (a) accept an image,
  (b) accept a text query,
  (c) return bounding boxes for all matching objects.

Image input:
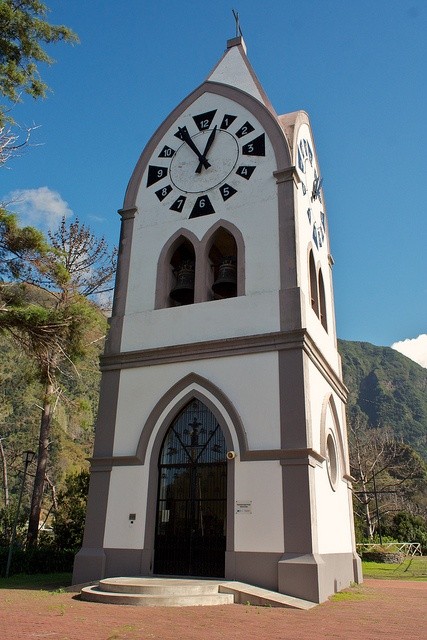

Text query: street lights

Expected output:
[5,449,35,575]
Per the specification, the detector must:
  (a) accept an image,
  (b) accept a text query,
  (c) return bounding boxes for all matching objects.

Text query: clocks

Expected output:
[145,109,266,219]
[296,138,326,250]
